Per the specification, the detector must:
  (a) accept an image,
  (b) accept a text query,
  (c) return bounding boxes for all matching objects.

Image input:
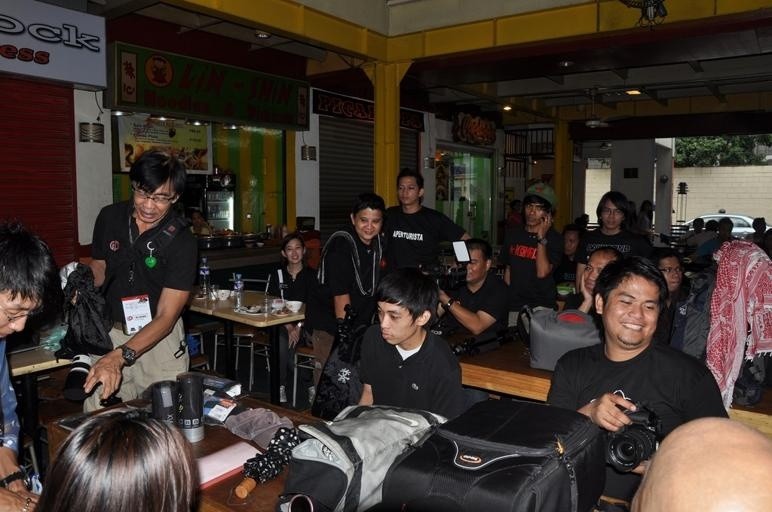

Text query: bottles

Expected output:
[234,274,245,309]
[199,258,210,289]
[266,224,271,240]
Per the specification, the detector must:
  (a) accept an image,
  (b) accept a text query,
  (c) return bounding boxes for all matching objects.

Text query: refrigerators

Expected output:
[184,175,237,231]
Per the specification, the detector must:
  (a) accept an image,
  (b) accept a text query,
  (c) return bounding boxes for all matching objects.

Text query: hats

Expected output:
[525,181,559,208]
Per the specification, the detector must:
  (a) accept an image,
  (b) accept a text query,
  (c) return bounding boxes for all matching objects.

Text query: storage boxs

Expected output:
[585,88,601,128]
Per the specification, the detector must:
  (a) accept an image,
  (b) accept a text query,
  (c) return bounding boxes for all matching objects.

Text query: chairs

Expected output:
[213,273,272,385]
[292,346,319,408]
[248,334,270,392]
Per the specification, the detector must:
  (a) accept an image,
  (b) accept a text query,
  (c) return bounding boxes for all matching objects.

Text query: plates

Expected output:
[234,306,276,315]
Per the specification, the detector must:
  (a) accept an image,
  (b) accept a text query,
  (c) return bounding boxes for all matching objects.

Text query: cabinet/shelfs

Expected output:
[197,239,281,293]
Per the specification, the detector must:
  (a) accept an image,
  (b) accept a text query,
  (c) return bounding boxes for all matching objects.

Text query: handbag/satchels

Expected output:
[265,270,312,346]
[277,405,447,512]
[529,309,605,371]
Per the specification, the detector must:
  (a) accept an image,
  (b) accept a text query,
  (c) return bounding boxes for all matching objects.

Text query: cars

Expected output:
[673,214,772,238]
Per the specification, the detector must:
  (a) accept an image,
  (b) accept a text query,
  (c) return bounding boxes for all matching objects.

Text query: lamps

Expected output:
[79,122,105,144]
[424,157,434,169]
[301,145,316,160]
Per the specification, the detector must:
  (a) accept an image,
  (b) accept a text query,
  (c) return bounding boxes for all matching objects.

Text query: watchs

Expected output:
[296,323,304,328]
[115,342,137,367]
[440,297,455,311]
[536,237,548,245]
[1,470,28,487]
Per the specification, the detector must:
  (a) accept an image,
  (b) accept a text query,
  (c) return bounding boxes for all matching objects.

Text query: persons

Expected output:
[0,221,59,512]
[499,182,564,312]
[548,256,733,475]
[506,199,522,225]
[565,245,621,316]
[269,231,316,404]
[746,218,767,250]
[651,248,713,357]
[70,149,199,413]
[685,220,718,245]
[636,200,660,238]
[357,268,465,426]
[562,224,584,280]
[630,416,771,512]
[581,214,589,223]
[678,218,704,246]
[625,199,639,233]
[310,189,396,393]
[189,210,210,235]
[432,237,510,351]
[384,166,470,270]
[37,408,203,512]
[574,217,590,235]
[688,217,733,268]
[575,190,649,293]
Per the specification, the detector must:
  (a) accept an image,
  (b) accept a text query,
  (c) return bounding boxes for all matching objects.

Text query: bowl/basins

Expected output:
[244,240,257,248]
[217,290,230,300]
[285,301,303,313]
[271,299,286,310]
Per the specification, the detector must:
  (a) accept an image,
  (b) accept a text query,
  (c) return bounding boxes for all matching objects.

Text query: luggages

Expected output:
[382,398,607,512]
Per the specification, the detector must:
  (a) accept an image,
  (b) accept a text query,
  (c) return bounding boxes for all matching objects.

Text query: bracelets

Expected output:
[22,497,31,512]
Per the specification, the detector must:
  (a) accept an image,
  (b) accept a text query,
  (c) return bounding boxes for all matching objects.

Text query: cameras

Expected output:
[65,352,94,401]
[599,398,661,476]
[418,240,472,295]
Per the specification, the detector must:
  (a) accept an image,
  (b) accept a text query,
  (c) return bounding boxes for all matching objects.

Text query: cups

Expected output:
[556,286,573,296]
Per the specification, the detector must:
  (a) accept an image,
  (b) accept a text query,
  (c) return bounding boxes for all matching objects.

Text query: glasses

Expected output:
[584,264,602,274]
[1,302,44,322]
[129,183,177,204]
[601,207,622,215]
[659,267,683,273]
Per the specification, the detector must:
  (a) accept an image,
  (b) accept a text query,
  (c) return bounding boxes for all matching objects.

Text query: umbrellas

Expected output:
[233,426,303,499]
